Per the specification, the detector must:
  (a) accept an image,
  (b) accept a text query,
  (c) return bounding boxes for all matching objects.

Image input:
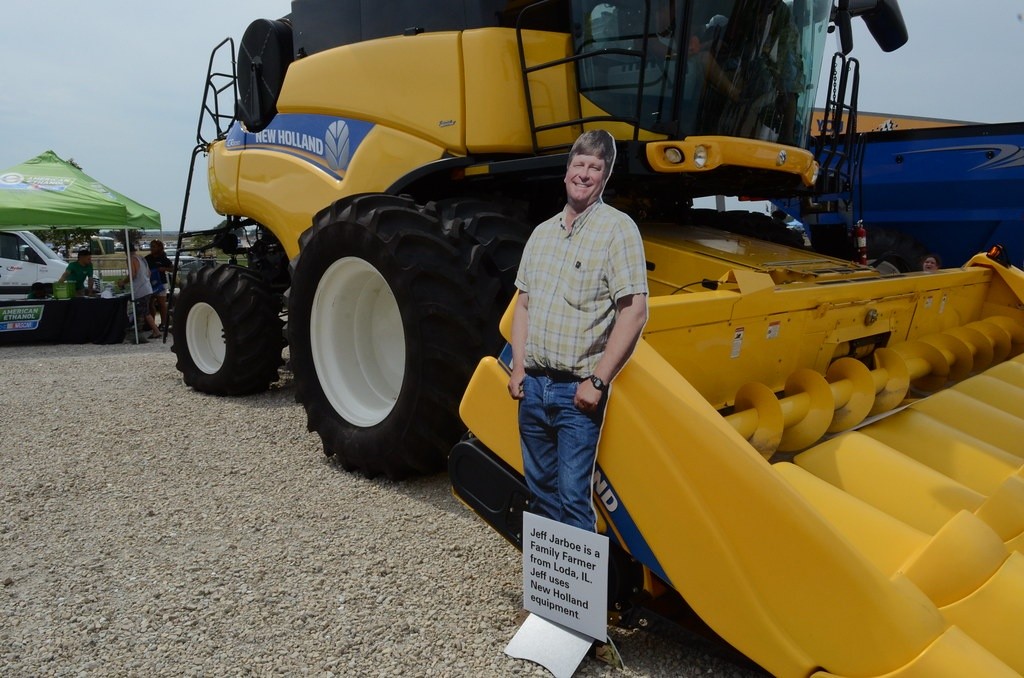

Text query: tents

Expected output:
[0,151,164,345]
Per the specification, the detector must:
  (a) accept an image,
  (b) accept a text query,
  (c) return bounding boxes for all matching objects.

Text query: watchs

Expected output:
[589,373,608,392]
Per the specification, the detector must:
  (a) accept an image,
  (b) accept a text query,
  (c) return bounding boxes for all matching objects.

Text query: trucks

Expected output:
[0,229,101,303]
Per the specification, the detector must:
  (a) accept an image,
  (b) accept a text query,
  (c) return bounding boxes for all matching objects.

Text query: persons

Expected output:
[772,209,789,224]
[117,240,176,341]
[648,0,745,105]
[57,250,96,299]
[921,256,943,273]
[508,129,648,529]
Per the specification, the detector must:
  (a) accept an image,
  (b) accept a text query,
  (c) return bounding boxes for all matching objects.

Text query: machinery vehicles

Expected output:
[156,2,1024,678]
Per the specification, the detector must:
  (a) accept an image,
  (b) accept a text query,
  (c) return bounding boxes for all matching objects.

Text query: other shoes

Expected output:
[126,327,135,332]
[145,326,152,331]
[148,334,162,339]
[159,325,166,332]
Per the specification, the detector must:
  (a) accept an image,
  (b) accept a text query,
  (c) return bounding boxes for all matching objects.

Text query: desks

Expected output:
[0,292,136,348]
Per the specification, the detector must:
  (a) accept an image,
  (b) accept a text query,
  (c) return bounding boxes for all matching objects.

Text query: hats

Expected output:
[78,246,92,256]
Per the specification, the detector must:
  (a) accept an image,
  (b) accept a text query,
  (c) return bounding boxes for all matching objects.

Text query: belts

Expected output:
[524,366,582,383]
